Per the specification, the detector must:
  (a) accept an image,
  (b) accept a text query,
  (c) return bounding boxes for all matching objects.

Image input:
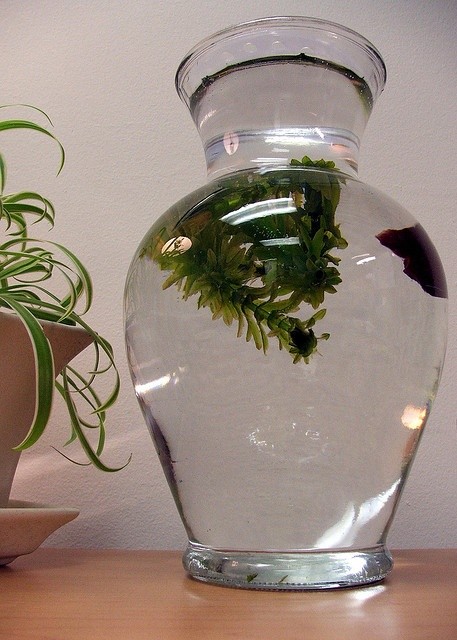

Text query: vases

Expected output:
[121,11,451,594]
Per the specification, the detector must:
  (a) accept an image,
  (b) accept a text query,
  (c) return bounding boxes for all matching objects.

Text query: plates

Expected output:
[1,501,88,572]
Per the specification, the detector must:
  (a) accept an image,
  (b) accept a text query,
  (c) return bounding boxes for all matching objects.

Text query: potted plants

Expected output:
[0,100,133,506]
